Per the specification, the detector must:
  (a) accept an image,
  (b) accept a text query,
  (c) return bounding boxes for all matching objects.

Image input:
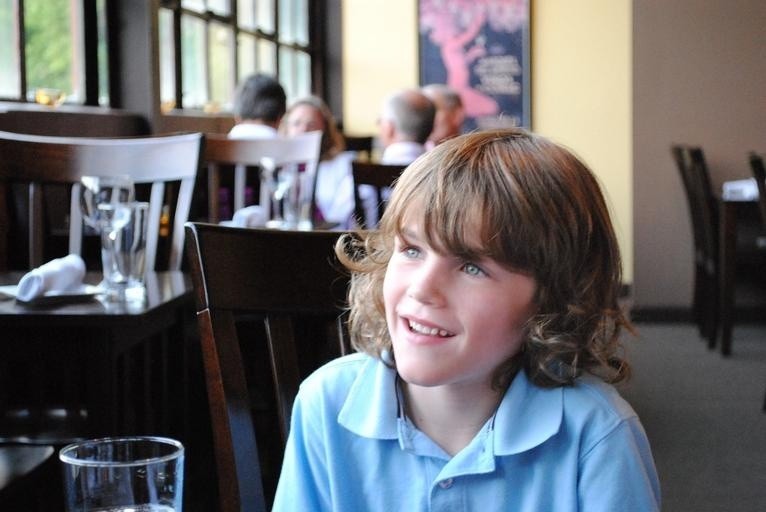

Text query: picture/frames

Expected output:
[415,1,534,138]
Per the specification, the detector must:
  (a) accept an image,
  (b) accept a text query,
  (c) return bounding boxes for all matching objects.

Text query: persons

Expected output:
[357,90,435,228]
[417,84,465,151]
[271,128,663,512]
[278,99,356,228]
[205,77,327,228]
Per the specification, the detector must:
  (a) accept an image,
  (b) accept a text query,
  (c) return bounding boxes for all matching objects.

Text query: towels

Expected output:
[17,254,87,303]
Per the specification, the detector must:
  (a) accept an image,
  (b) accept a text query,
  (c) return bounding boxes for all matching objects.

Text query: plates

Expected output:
[0,285,103,300]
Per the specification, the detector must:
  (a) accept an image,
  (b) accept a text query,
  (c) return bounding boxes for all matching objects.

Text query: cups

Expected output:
[96,203,150,301]
[58,435,187,511]
[80,172,133,287]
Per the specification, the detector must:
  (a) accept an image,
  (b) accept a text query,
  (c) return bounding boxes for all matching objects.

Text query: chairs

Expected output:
[669,148,766,355]
[201,129,324,220]
[348,161,410,225]
[0,105,152,240]
[187,219,415,510]
[2,132,209,272]
